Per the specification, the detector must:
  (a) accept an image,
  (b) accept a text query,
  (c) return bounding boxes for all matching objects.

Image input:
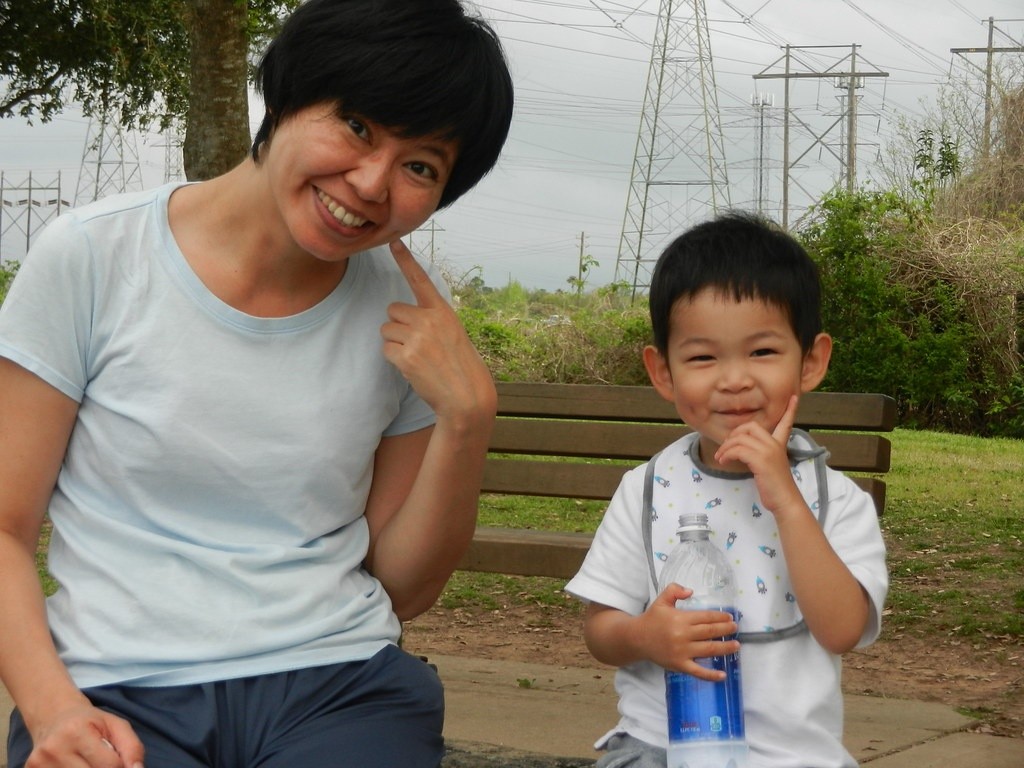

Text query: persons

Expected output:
[0,0,516,768]
[561,209,891,768]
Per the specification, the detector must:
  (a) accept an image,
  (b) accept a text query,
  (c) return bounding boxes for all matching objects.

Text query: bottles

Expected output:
[659,514,749,768]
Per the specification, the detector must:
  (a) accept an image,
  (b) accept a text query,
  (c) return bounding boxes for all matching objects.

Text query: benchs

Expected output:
[457,381,898,585]
